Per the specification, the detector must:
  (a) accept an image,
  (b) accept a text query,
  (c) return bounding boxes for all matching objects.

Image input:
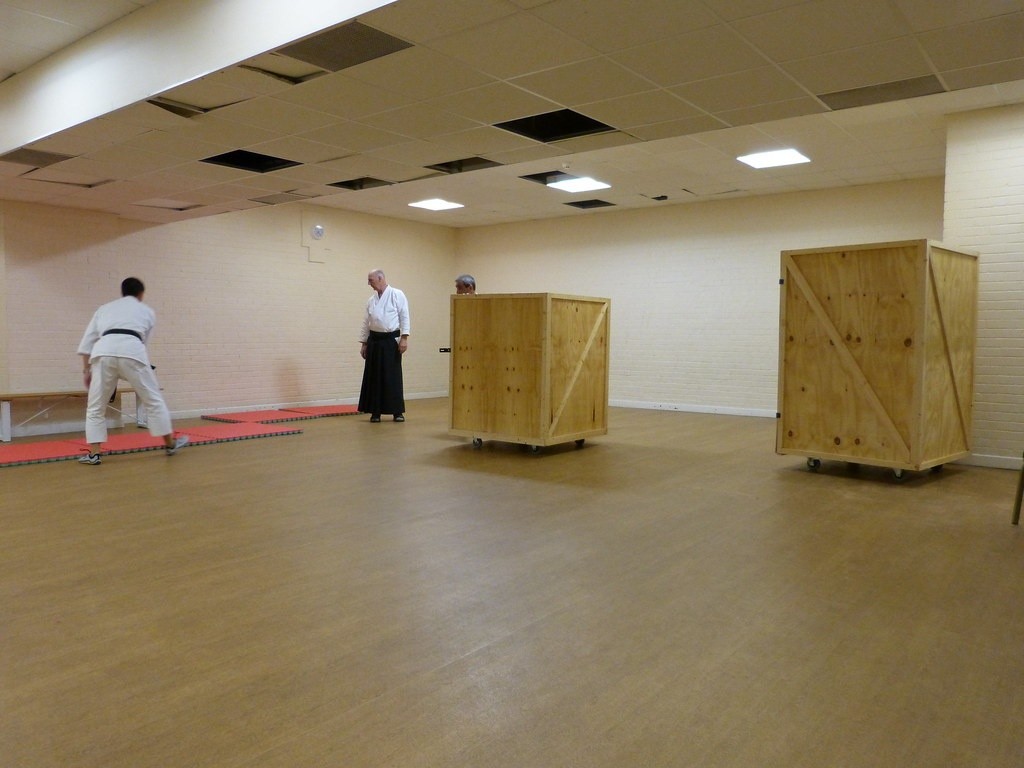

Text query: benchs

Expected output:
[0,386,165,443]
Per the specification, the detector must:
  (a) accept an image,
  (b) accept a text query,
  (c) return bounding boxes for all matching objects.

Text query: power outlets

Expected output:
[438,347,451,354]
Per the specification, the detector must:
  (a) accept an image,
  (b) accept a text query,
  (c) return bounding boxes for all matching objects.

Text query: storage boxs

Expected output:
[774,237,979,480]
[446,291,614,456]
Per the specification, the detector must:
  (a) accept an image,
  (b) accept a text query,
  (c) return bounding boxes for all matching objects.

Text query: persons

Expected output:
[359,270,410,422]
[455,274,476,294]
[77,277,188,464]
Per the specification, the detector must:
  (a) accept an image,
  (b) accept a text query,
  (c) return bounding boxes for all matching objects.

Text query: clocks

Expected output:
[313,225,324,240]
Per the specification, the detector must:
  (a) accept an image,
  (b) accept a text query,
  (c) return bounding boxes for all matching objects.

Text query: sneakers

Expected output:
[166,436,188,455]
[78,448,100,465]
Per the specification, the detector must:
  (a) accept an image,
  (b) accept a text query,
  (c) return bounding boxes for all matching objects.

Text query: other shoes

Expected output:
[394,414,405,422]
[371,416,380,422]
